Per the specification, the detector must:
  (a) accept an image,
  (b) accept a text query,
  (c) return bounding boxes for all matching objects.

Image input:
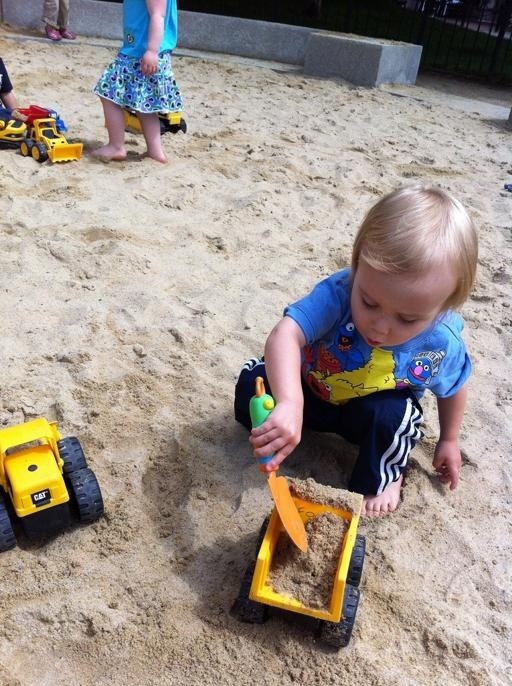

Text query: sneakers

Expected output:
[44,23,77,41]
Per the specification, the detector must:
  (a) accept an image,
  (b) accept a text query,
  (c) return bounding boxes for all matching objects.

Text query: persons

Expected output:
[0,55,20,118]
[41,0,76,41]
[89,0,179,163]
[231,182,479,515]
